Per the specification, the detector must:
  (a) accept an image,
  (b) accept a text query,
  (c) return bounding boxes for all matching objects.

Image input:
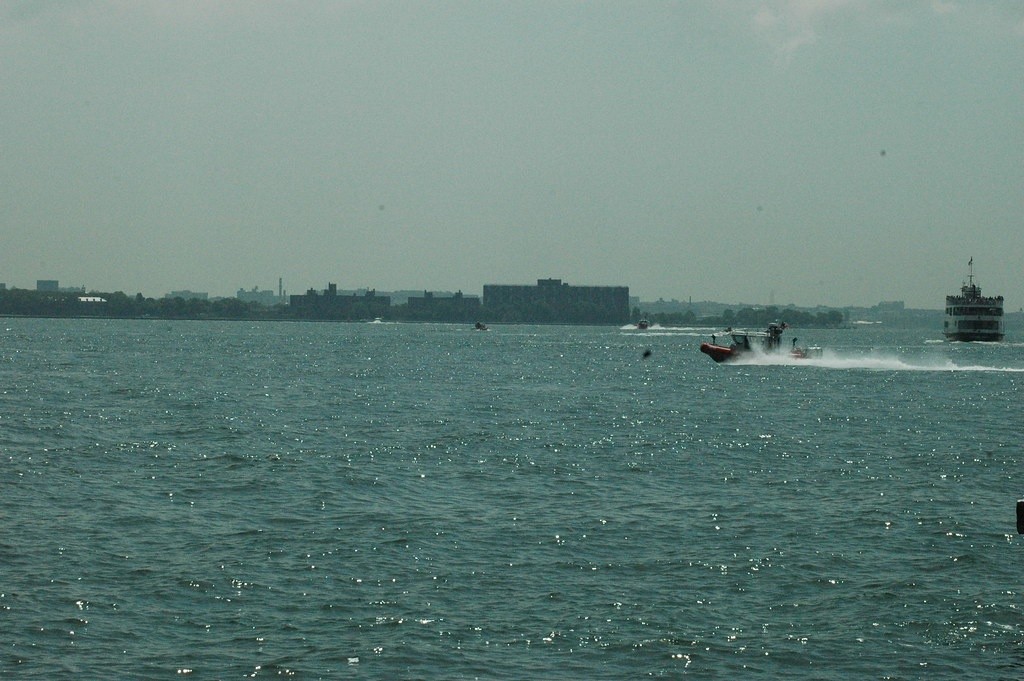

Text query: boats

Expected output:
[636,319,648,330]
[941,275,1006,342]
[700,322,807,364]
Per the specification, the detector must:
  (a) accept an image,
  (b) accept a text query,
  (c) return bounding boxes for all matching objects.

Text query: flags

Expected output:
[968,258,972,265]
